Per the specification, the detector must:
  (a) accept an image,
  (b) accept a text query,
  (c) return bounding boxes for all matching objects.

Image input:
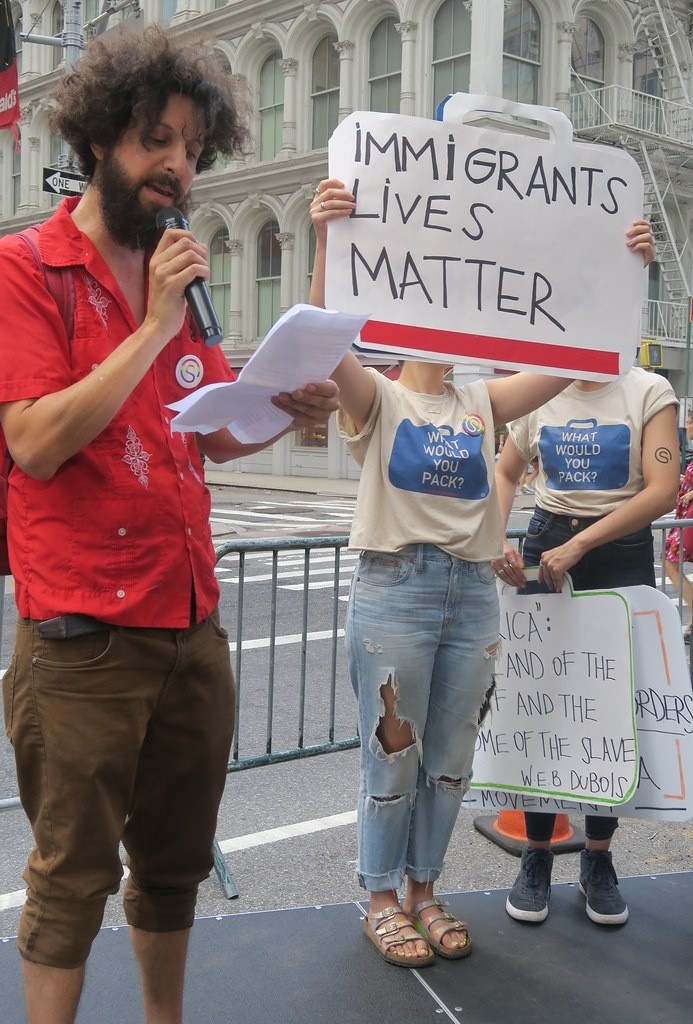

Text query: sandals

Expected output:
[359,906,435,968]
[400,897,473,961]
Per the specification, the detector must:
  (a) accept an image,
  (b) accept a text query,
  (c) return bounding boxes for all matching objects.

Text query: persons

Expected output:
[309,177,656,968]
[657,411,693,644]
[491,366,682,925]
[0,38,341,1024]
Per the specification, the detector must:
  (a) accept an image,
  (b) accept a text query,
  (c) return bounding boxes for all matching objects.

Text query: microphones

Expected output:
[156,205,223,347]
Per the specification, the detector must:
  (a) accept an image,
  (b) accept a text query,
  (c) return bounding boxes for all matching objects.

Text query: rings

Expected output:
[502,564,508,570]
[498,569,503,574]
[508,558,515,564]
[315,187,320,194]
[539,561,543,567]
[321,202,325,210]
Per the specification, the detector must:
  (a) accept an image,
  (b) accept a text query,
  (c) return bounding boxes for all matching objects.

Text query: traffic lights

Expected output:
[645,344,663,367]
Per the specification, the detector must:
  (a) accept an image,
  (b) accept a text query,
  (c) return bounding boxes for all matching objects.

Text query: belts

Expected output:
[38,614,128,641]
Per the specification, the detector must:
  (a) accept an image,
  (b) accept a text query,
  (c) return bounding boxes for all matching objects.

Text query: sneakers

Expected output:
[578,849,629,924]
[505,846,554,922]
